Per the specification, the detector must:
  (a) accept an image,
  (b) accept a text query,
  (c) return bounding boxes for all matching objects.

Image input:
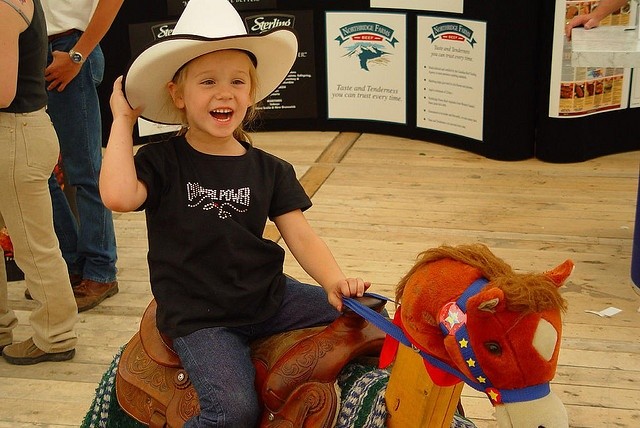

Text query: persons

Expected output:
[0,1,79,366]
[41,1,123,311]
[97,48,372,427]
[565,1,628,42]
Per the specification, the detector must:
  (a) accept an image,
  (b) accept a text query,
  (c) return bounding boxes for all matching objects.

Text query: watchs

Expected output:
[69,50,86,64]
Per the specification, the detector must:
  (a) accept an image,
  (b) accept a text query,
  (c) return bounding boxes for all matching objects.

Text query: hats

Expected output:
[122,0,299,125]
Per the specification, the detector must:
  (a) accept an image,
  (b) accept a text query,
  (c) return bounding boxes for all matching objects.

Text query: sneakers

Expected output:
[74,278,118,313]
[24,273,74,300]
[0,345,12,354]
[3,336,76,364]
